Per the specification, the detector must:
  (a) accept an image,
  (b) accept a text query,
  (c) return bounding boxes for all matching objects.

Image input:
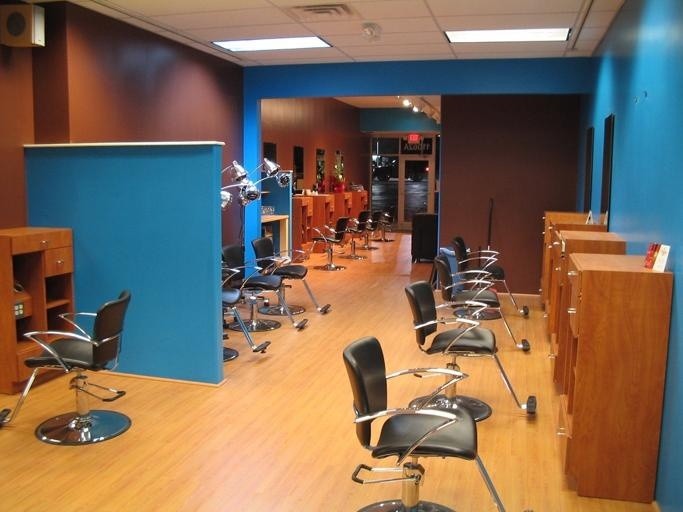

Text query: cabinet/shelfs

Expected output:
[290,190,368,263]
[550,229,626,395]
[557,253,672,504]
[539,211,607,314]
[0,226,76,396]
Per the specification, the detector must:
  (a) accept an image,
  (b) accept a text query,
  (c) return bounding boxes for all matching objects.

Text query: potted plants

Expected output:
[331,150,348,194]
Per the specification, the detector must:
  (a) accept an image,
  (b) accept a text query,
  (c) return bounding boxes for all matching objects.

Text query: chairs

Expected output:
[404,280,536,421]
[1,290,133,447]
[223,236,333,361]
[304,203,395,271]
[452,235,529,316]
[434,255,531,352]
[342,335,508,512]
